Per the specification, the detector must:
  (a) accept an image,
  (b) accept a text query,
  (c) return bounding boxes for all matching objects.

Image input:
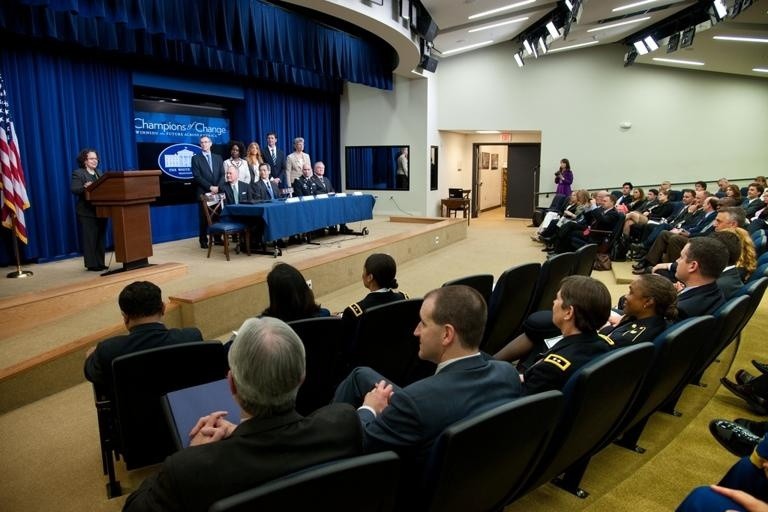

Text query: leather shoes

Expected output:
[214,240,229,246]
[708,419,761,458]
[88,267,102,271]
[328,229,339,235]
[535,236,540,241]
[632,267,646,274]
[735,369,757,385]
[735,418,768,438]
[277,239,286,248]
[340,227,353,234]
[201,242,208,248]
[632,243,644,252]
[633,254,642,259]
[530,234,539,241]
[721,377,768,415]
[97,265,108,269]
[632,259,653,269]
[751,359,768,374]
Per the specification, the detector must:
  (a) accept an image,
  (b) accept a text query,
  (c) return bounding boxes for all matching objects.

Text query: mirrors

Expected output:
[345,145,410,191]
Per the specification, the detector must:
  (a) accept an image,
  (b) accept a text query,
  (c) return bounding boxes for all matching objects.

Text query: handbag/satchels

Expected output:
[556,216,571,227]
[593,254,611,270]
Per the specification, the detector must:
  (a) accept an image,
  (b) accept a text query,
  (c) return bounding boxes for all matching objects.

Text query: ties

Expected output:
[232,185,238,204]
[320,178,323,183]
[271,149,276,166]
[206,155,213,173]
[266,182,273,198]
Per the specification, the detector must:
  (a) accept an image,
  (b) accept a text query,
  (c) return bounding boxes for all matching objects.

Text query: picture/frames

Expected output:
[482,152,490,169]
[491,154,498,169]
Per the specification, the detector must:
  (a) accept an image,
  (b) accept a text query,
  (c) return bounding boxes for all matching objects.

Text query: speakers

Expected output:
[419,56,438,73]
[418,14,437,42]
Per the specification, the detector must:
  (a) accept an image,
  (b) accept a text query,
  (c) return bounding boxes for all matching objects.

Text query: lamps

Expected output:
[514,0,583,67]
[367,0,442,73]
[624,0,753,66]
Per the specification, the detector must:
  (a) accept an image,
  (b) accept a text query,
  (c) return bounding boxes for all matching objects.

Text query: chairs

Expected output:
[757,252,768,266]
[589,212,626,253]
[739,277,768,330]
[514,342,656,499]
[288,318,350,415]
[750,263,768,279]
[448,188,464,218]
[751,229,764,240]
[522,252,582,333]
[572,244,598,277]
[442,274,494,303]
[754,235,767,258]
[406,391,563,512]
[208,450,399,512]
[355,298,423,367]
[696,294,751,390]
[200,193,251,260]
[603,315,716,454]
[483,263,541,354]
[96,340,225,499]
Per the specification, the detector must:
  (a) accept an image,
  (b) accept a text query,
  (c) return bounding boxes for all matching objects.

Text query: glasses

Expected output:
[87,158,99,161]
[200,141,209,144]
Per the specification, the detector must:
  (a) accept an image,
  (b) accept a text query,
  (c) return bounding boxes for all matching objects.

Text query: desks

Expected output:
[220,191,376,258]
[441,198,470,225]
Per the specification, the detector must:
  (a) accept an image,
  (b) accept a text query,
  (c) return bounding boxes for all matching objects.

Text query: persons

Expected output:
[524,275,616,389]
[262,131,285,189]
[71,149,108,270]
[624,187,646,211]
[491,274,680,373]
[661,181,671,193]
[543,192,599,251]
[192,136,226,248]
[623,190,673,242]
[549,195,620,256]
[223,140,251,184]
[256,262,334,331]
[548,191,609,254]
[720,369,768,416]
[286,137,314,186]
[616,231,745,310]
[293,164,326,236]
[555,158,573,195]
[85,281,204,387]
[754,176,764,186]
[245,142,266,181]
[695,181,707,192]
[751,190,768,220]
[615,182,633,207]
[312,162,353,233]
[124,318,364,512]
[219,166,262,249]
[725,185,740,199]
[709,413,768,458]
[397,146,412,191]
[333,285,524,451]
[628,189,696,258]
[718,178,730,191]
[530,191,578,242]
[651,206,747,285]
[750,358,768,374]
[716,198,736,208]
[538,191,588,243]
[343,254,411,328]
[253,164,303,248]
[633,196,719,273]
[740,183,764,218]
[674,227,756,283]
[709,485,768,511]
[609,236,726,327]
[636,189,658,213]
[629,191,710,261]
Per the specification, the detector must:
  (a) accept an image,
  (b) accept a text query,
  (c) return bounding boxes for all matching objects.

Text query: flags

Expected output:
[0,74,31,244]
